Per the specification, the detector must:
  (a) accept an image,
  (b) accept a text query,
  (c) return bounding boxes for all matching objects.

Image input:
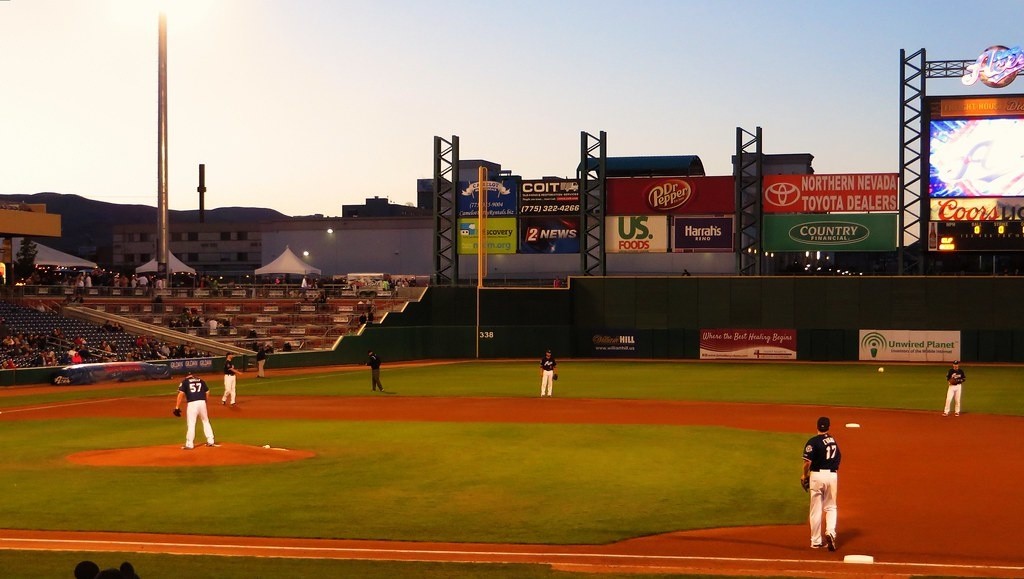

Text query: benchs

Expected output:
[0,301,205,370]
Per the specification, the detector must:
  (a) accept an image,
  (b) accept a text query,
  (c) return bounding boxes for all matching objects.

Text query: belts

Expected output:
[810,469,836,472]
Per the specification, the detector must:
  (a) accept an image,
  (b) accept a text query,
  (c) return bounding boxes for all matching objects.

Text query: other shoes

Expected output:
[181,446,192,450]
[825,534,835,552]
[380,388,384,392]
[954,412,959,416]
[812,545,821,552]
[207,443,220,447]
[942,413,949,416]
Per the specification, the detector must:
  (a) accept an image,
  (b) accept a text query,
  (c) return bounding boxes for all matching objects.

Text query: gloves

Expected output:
[173,408,181,417]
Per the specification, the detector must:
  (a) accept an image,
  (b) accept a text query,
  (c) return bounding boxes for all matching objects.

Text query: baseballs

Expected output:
[878,367,884,373]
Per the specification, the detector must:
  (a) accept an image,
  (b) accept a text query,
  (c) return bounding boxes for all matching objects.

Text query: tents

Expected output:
[16,239,98,267]
[255,248,321,283]
[136,250,196,289]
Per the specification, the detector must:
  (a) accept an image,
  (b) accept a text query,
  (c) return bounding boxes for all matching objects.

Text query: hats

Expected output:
[953,360,958,364]
[226,352,233,356]
[546,350,551,353]
[817,417,829,431]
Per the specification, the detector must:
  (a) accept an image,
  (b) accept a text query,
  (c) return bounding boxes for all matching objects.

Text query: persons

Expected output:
[0,317,90,369]
[365,351,384,391]
[169,317,200,332]
[802,417,841,551]
[250,330,259,352]
[539,350,558,397]
[205,317,230,327]
[352,277,417,289]
[1003,268,1019,276]
[0,268,224,298]
[241,272,327,303]
[942,360,966,416]
[266,343,291,354]
[257,348,266,378]
[222,353,241,406]
[360,310,373,324]
[74,559,139,579]
[174,372,215,449]
[554,276,567,288]
[682,269,690,276]
[100,320,214,361]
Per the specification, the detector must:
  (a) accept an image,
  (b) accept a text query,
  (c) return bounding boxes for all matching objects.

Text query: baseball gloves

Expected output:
[553,374,559,380]
[950,378,957,385]
[174,408,182,417]
[800,476,810,491]
